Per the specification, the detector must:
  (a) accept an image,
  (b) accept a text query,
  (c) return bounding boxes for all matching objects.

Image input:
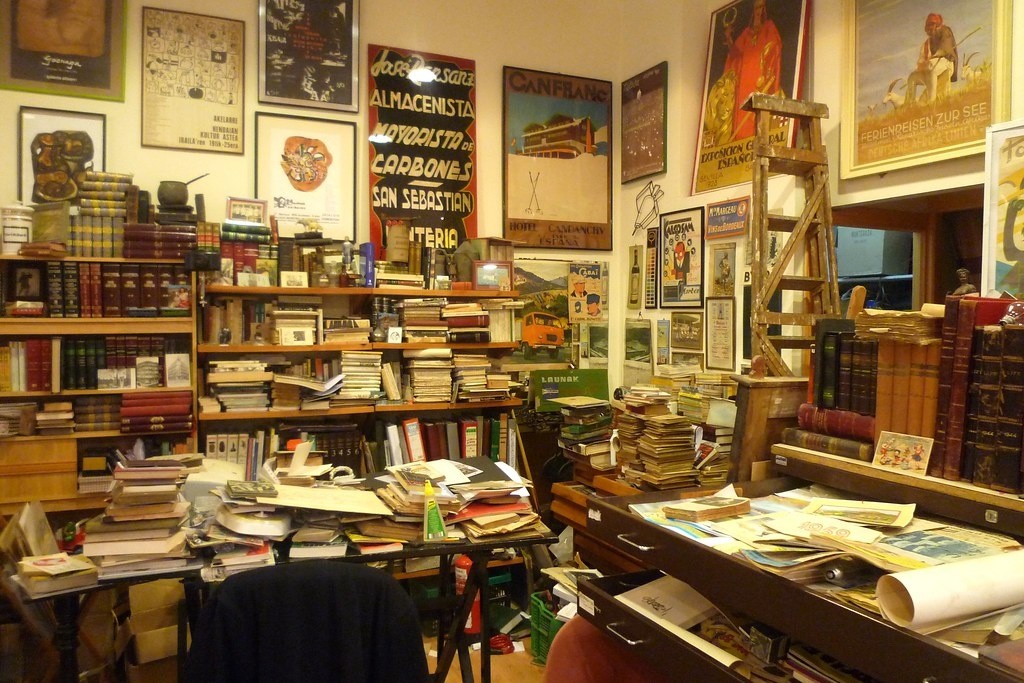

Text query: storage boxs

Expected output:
[529,590,572,663]
[420,571,514,636]
[126,578,193,666]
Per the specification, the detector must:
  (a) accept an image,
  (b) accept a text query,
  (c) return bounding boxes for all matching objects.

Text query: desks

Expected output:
[0,477,558,683]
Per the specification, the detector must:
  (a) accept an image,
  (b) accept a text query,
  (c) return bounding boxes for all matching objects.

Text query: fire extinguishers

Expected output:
[455,554,482,635]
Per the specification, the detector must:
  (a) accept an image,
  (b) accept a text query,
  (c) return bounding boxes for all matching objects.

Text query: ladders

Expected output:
[740,92,841,378]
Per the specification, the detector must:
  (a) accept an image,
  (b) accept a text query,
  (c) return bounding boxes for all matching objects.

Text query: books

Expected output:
[663,496,751,522]
[0,169,195,436]
[782,295,1024,494]
[977,637,1024,681]
[195,207,524,413]
[540,567,604,622]
[545,363,738,490]
[0,447,204,601]
[782,641,875,683]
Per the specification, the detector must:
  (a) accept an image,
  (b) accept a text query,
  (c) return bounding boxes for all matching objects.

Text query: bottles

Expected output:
[339,266,349,287]
[629,250,641,304]
[158,181,188,205]
[423,478,450,543]
[822,560,868,587]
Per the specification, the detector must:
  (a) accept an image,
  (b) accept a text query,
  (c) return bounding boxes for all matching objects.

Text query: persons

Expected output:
[952,268,977,294]
[201,413,550,572]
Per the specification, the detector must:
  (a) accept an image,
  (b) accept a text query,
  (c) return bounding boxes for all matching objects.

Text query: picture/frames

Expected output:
[670,311,703,349]
[838,0,1011,183]
[670,351,704,372]
[502,65,612,252]
[659,206,704,310]
[981,116,1024,297]
[0,0,128,103]
[258,0,360,111]
[253,112,358,242]
[140,6,246,154]
[690,0,808,194]
[17,105,107,207]
[707,242,737,298]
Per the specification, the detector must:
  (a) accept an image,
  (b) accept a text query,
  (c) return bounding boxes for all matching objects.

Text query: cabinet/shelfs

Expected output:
[0,254,523,529]
[584,465,1024,683]
